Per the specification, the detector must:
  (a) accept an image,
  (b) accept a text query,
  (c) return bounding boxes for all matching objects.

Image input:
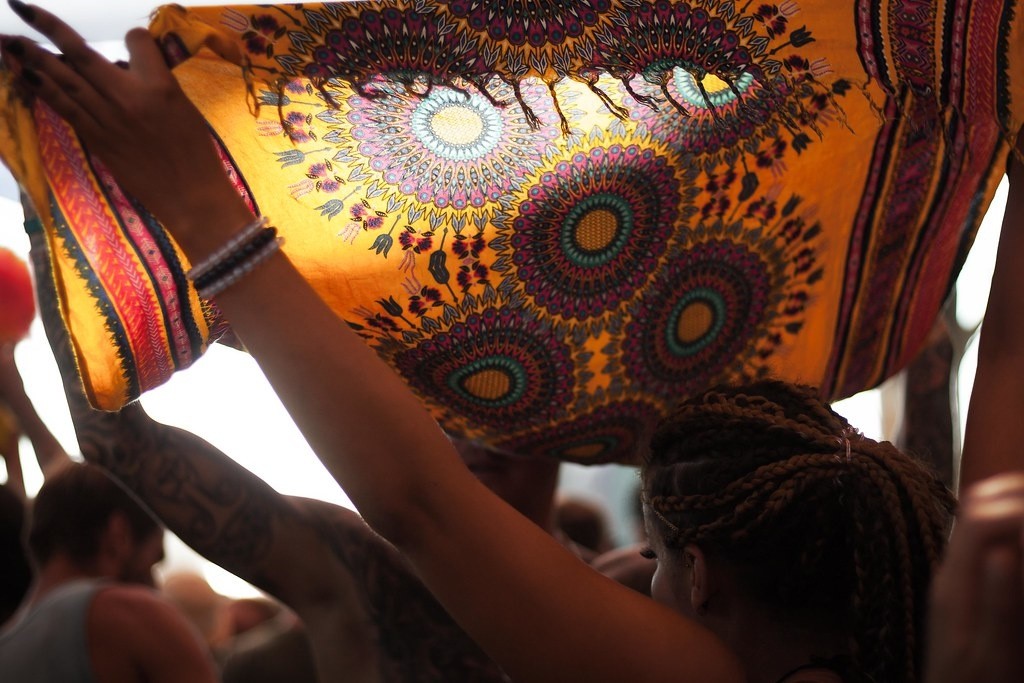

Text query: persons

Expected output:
[1,348,660,683]
[0,1,1024,683]
[927,474,1023,683]
[19,181,660,683]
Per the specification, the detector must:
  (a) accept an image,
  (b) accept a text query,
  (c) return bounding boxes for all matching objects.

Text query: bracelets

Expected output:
[186,216,284,301]
